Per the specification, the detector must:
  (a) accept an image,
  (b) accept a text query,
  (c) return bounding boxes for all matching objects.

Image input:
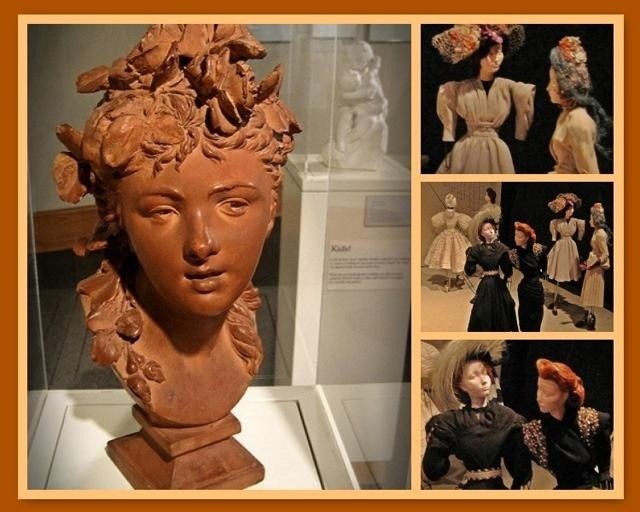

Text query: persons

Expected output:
[333,38,388,151]
[50,24,303,430]
